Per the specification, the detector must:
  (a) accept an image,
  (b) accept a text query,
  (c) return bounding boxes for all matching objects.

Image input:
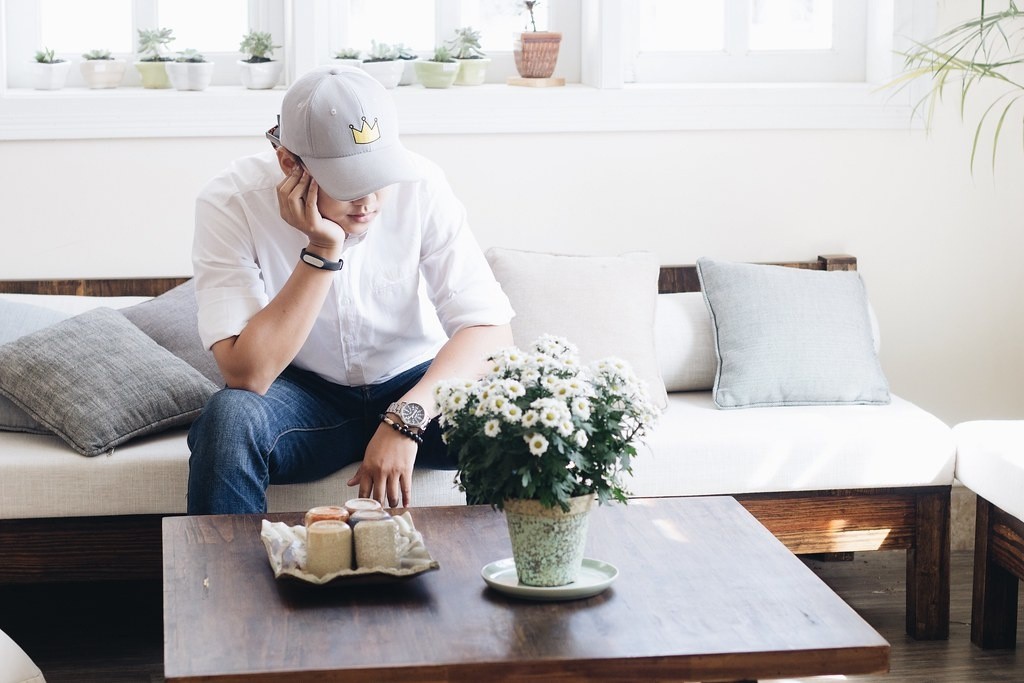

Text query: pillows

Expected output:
[0,306,220,455]
[695,255,891,408]
[483,246,667,410]
[117,273,229,394]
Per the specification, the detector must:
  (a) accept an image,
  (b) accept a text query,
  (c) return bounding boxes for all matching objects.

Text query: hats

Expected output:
[277,65,432,202]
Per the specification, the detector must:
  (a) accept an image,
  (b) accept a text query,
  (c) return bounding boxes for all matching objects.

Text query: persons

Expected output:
[186,63,517,516]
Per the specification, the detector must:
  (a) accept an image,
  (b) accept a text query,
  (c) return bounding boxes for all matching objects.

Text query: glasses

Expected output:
[265,125,303,171]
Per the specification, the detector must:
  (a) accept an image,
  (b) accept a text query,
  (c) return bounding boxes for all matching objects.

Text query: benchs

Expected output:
[0,261,963,642]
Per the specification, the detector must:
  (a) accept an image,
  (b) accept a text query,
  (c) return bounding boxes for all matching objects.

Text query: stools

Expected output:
[953,418,1024,648]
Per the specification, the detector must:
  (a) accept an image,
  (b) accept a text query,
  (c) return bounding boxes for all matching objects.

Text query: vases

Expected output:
[499,484,596,588]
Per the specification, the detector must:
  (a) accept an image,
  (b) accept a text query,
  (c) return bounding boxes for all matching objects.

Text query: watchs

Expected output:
[385,401,432,436]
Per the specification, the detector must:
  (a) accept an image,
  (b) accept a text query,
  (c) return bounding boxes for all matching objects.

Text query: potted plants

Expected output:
[132,27,175,89]
[363,40,403,90]
[31,46,72,93]
[512,1,561,77]
[236,29,284,89]
[415,45,460,89]
[175,47,214,91]
[333,46,363,68]
[447,26,490,86]
[393,44,415,87]
[81,48,125,89]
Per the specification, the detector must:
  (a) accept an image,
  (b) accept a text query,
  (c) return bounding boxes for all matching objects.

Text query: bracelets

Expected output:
[299,248,344,271]
[377,413,424,446]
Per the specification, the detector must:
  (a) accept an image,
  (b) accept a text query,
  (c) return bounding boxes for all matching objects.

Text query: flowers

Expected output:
[437,333,659,513]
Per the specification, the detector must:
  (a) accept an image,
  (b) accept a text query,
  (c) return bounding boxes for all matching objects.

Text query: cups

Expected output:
[306,499,401,579]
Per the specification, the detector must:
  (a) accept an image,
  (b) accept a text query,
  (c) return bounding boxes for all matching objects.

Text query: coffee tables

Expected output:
[161,495,892,683]
